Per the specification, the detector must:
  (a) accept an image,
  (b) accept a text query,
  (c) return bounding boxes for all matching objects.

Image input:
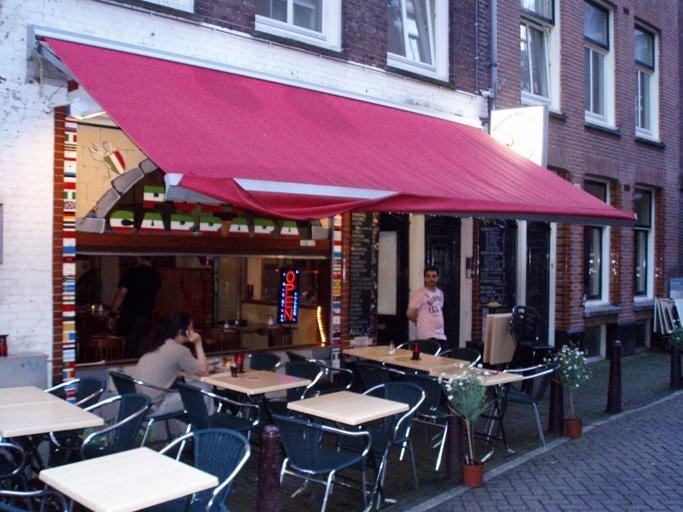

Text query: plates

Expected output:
[214,361,232,370]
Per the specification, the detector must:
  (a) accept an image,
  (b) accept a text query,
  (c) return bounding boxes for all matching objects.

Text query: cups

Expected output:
[229,365,238,378]
[411,341,420,359]
[233,352,243,373]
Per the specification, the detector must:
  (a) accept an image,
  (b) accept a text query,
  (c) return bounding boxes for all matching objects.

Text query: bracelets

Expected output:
[109,311,117,318]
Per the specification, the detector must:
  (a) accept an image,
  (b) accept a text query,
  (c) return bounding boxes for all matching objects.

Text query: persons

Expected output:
[133,308,215,417]
[108,256,161,358]
[406,267,447,354]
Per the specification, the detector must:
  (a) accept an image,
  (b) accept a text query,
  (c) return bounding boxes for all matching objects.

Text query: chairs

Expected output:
[75,288,586,509]
[41,391,153,512]
[27,375,109,470]
[0,442,71,511]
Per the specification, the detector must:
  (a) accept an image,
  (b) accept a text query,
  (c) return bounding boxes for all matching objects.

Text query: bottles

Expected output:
[387,344,395,355]
[223,321,229,329]
[267,313,274,325]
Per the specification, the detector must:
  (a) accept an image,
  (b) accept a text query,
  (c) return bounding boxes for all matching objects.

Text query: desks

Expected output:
[0,384,104,445]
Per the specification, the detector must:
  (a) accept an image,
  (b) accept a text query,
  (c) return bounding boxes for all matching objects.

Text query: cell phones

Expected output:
[179,328,187,336]
